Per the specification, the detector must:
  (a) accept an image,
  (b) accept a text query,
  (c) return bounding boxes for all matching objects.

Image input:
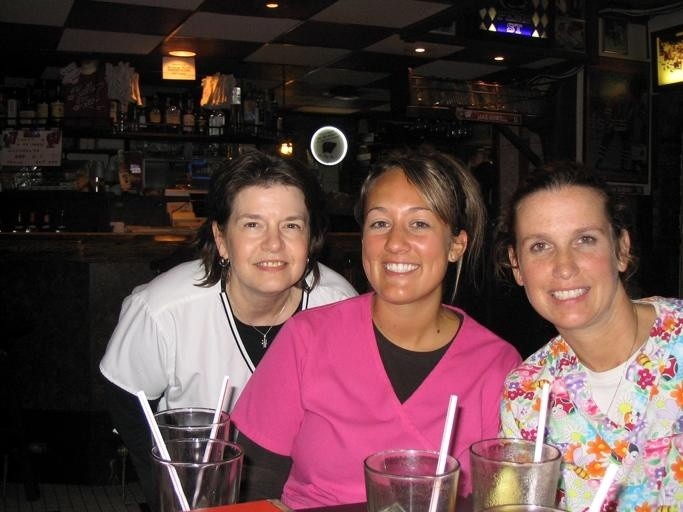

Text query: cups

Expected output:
[146,437,242,512]
[469,436,564,512]
[148,408,230,511]
[363,448,460,512]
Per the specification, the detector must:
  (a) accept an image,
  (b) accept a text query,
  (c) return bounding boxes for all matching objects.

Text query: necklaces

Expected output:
[226,284,289,349]
[554,307,639,424]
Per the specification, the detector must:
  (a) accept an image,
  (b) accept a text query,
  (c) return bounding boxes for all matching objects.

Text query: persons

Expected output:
[497,163,683,512]
[95,151,358,512]
[230,151,523,512]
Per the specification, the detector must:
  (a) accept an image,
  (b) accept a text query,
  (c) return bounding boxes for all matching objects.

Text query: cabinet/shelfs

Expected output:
[2,126,278,231]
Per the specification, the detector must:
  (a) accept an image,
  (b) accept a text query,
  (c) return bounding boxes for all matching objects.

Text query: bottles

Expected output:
[110,85,281,139]
[4,83,65,129]
[11,205,72,233]
